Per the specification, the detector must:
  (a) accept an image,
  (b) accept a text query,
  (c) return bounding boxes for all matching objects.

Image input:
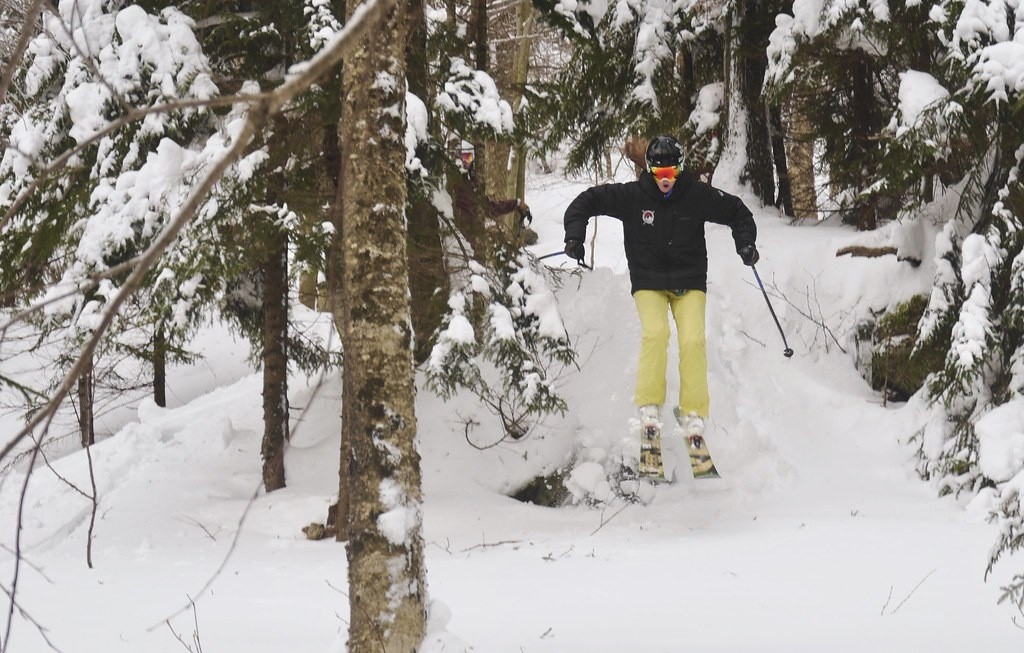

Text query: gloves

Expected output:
[736,242,759,266]
[564,239,585,259]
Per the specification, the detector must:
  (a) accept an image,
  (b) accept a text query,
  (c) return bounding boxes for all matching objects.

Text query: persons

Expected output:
[563,136,759,448]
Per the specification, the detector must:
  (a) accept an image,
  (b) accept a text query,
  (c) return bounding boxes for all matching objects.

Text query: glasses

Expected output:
[650,166,681,180]
[461,153,472,163]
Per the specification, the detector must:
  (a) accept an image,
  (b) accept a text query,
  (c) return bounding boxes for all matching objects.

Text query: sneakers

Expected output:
[638,404,659,442]
[687,417,704,449]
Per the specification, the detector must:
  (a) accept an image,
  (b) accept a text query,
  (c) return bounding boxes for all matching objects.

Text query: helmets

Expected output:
[645,135,684,166]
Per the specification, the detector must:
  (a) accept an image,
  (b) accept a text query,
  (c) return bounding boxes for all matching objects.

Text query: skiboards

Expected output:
[635,404,722,484]
